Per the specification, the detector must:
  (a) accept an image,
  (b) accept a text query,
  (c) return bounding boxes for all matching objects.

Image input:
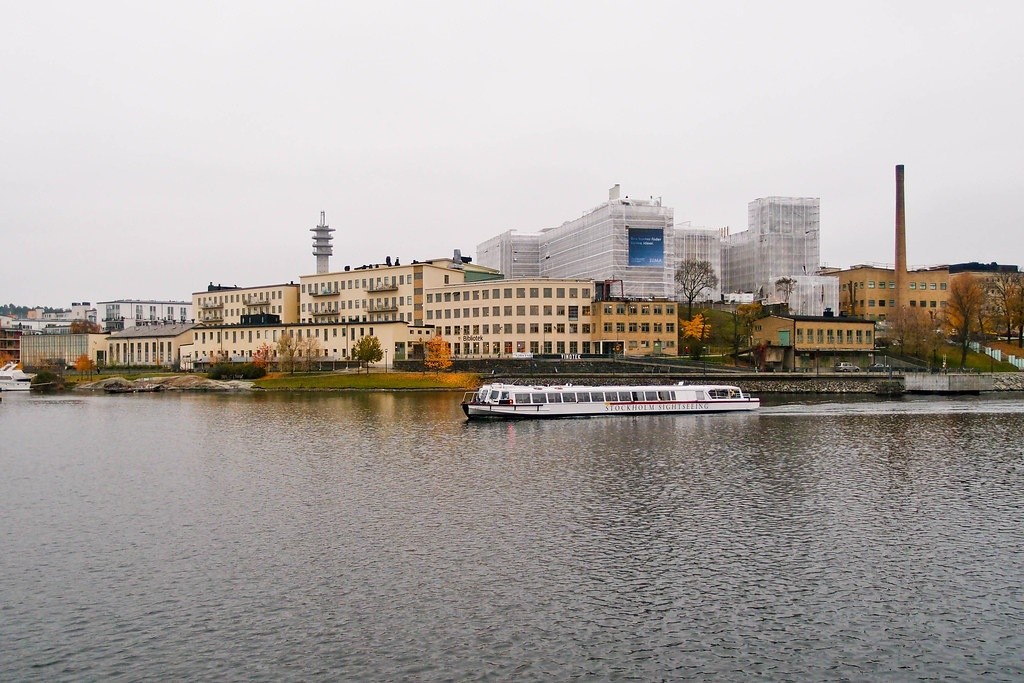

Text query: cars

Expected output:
[868,363,891,372]
[836,362,861,372]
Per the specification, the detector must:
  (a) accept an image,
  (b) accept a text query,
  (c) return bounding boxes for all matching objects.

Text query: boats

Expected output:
[461,383,760,419]
[0,363,34,390]
